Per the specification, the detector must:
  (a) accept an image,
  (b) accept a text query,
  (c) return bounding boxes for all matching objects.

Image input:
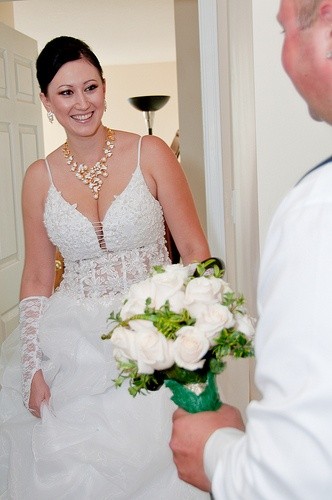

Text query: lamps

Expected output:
[127,95,170,136]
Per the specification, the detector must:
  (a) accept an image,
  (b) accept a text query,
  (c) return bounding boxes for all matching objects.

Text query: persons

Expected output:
[0,36,218,500]
[168,0,332,499]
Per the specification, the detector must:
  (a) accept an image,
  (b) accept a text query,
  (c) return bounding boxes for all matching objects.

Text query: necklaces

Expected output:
[63,128,114,195]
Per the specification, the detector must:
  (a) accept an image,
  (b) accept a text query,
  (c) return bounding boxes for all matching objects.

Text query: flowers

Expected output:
[100,259,257,414]
